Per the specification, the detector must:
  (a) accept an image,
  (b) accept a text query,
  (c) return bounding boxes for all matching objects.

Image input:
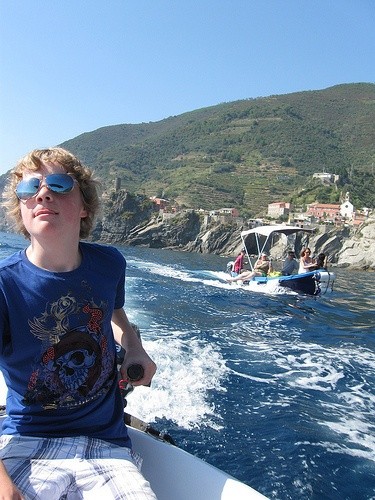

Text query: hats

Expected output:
[241,247,246,253]
[262,252,267,257]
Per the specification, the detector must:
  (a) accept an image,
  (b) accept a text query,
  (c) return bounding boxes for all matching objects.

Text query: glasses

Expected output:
[306,251,311,253]
[13,173,79,201]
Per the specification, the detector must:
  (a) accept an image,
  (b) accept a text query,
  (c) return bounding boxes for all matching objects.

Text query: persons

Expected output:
[278,249,299,278]
[0,146,159,500]
[308,253,329,271]
[225,251,270,283]
[232,248,248,274]
[297,248,317,274]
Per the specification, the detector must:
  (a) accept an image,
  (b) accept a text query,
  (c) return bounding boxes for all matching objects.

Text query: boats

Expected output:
[226,225,336,298]
